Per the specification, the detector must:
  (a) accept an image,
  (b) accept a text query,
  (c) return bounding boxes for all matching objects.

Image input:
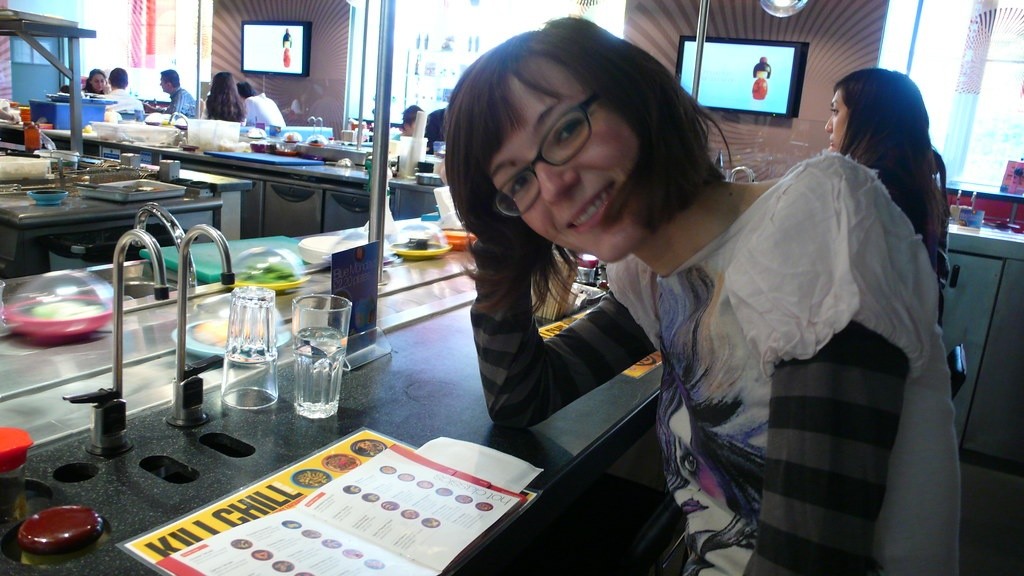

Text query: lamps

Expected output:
[760,0,807,17]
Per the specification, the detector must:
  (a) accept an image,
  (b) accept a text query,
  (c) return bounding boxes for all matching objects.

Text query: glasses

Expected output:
[490,86,604,220]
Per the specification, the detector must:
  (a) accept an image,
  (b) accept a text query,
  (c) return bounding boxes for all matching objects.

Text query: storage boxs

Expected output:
[28,98,108,128]
[187,119,240,150]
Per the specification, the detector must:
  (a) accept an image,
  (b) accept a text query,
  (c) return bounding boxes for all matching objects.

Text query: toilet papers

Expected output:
[405,110,428,175]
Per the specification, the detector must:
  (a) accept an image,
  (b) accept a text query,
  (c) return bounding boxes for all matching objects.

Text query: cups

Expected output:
[221,287,278,409]
[291,295,352,418]
[134,109,143,122]
[434,141,445,158]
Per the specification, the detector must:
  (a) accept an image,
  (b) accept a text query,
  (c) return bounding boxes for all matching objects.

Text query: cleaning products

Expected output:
[364,160,396,239]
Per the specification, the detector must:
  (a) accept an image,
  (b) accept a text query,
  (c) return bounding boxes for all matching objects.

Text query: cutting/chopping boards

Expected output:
[140,234,309,283]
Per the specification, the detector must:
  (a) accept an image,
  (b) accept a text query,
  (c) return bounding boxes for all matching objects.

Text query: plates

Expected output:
[290,469,333,489]
[276,150,297,155]
[28,191,67,205]
[2,296,113,335]
[435,234,476,250]
[146,122,161,126]
[172,321,291,353]
[322,455,361,473]
[175,125,187,130]
[391,243,453,257]
[307,142,326,145]
[284,141,298,143]
[350,439,387,457]
[224,275,311,289]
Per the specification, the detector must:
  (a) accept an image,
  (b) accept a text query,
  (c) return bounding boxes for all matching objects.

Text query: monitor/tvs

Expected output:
[239,21,312,77]
[675,35,810,118]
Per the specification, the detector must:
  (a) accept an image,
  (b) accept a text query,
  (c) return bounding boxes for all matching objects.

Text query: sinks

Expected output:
[75,259,214,299]
[141,144,179,150]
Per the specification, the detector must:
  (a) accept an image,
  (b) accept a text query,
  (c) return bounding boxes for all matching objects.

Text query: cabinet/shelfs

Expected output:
[942,250,1024,464]
[239,178,370,237]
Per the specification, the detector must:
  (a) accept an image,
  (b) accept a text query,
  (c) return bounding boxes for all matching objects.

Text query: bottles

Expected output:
[24,122,42,152]
[0,427,32,541]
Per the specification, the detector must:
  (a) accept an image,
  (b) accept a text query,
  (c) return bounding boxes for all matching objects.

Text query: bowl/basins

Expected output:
[298,237,339,264]
[251,143,270,153]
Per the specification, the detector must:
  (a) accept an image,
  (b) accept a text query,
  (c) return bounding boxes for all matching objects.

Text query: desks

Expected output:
[0,190,223,280]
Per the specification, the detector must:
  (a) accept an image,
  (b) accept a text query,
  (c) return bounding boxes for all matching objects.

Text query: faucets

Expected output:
[167,112,190,144]
[163,223,236,428]
[315,117,323,135]
[63,222,169,458]
[956,189,963,219]
[131,201,198,294]
[306,116,317,134]
[970,191,977,211]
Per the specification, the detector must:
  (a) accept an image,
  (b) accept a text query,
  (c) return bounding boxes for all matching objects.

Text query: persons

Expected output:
[391,105,447,155]
[824,68,944,329]
[236,81,286,129]
[438,17,961,576]
[200,72,248,127]
[81,67,197,121]
